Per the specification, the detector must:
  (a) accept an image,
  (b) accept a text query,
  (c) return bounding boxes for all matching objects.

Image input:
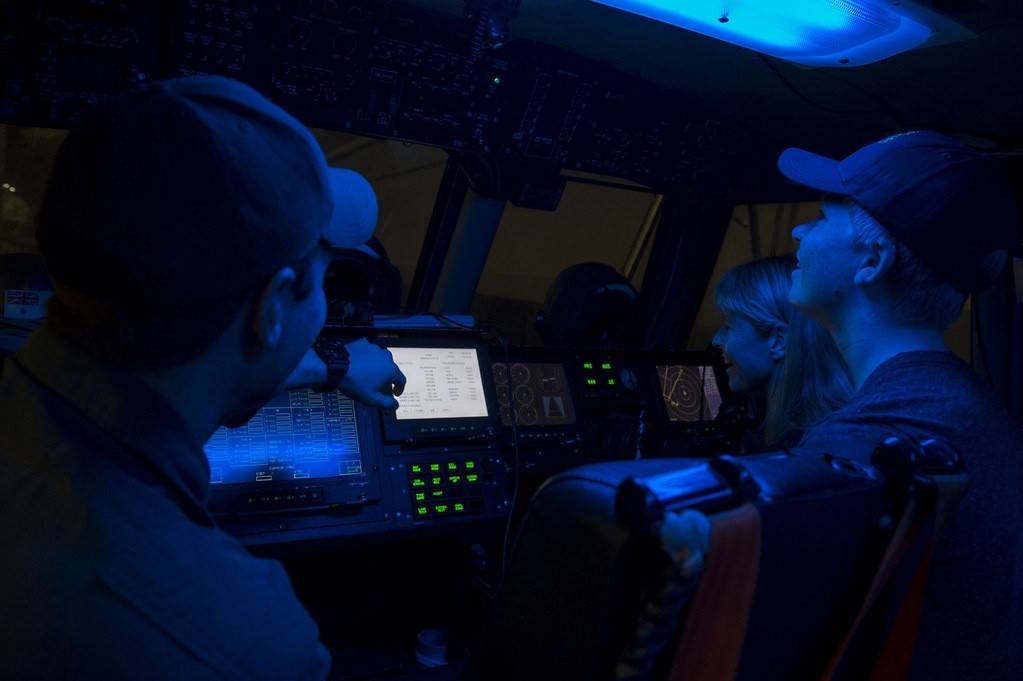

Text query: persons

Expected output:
[2,72,407,680]
[712,254,856,454]
[776,129,1023,679]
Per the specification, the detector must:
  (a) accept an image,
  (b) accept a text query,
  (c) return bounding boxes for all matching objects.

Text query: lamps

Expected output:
[590,0,978,67]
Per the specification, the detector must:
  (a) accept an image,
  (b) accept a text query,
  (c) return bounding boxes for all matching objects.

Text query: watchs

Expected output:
[311,337,350,392]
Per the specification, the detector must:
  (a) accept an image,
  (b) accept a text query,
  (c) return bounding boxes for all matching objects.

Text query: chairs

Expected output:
[465,436,973,681]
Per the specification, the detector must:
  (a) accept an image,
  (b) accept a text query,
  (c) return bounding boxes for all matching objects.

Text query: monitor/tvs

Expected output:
[373,335,495,443]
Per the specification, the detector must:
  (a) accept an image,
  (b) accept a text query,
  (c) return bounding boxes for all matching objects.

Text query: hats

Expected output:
[34,72,380,302]
[776,128,1018,296]
